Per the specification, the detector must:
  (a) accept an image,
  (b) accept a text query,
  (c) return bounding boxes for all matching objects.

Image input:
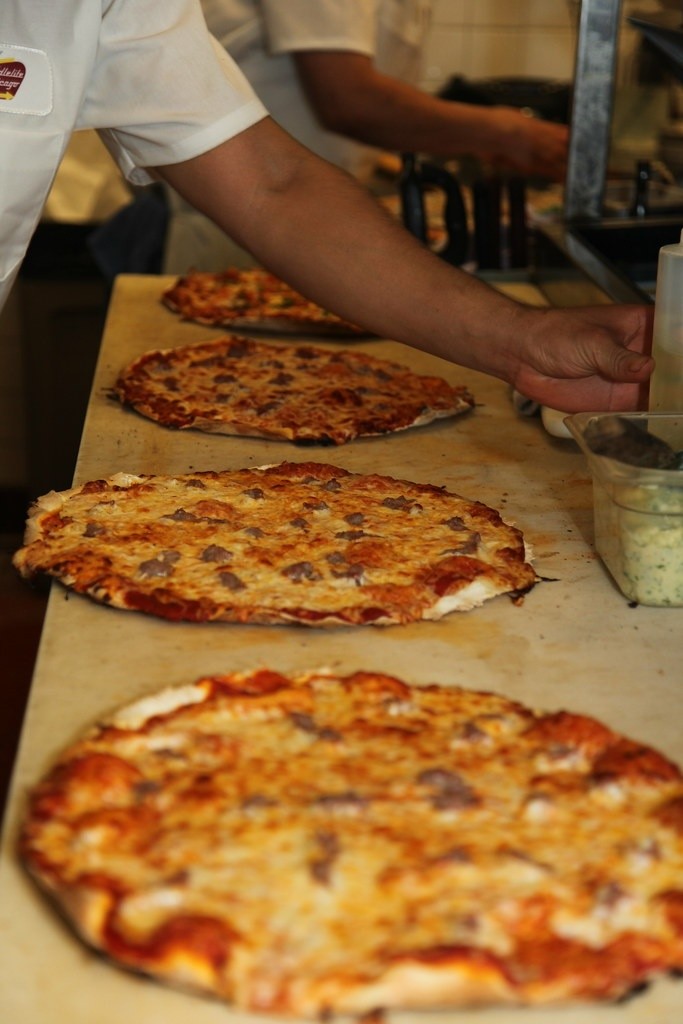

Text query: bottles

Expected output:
[647,230,683,452]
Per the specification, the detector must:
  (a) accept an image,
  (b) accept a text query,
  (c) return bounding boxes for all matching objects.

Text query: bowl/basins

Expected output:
[564,412,683,608]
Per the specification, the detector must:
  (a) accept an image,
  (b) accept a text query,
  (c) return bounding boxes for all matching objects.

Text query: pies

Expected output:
[159,267,383,339]
[14,460,545,631]
[107,335,473,447]
[22,667,681,1021]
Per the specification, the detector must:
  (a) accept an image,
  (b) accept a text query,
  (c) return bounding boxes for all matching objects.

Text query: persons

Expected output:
[196,1,575,288]
[0,1,655,414]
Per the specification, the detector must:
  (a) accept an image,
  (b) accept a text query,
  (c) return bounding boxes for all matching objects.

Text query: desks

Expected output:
[1,275,682,1022]
